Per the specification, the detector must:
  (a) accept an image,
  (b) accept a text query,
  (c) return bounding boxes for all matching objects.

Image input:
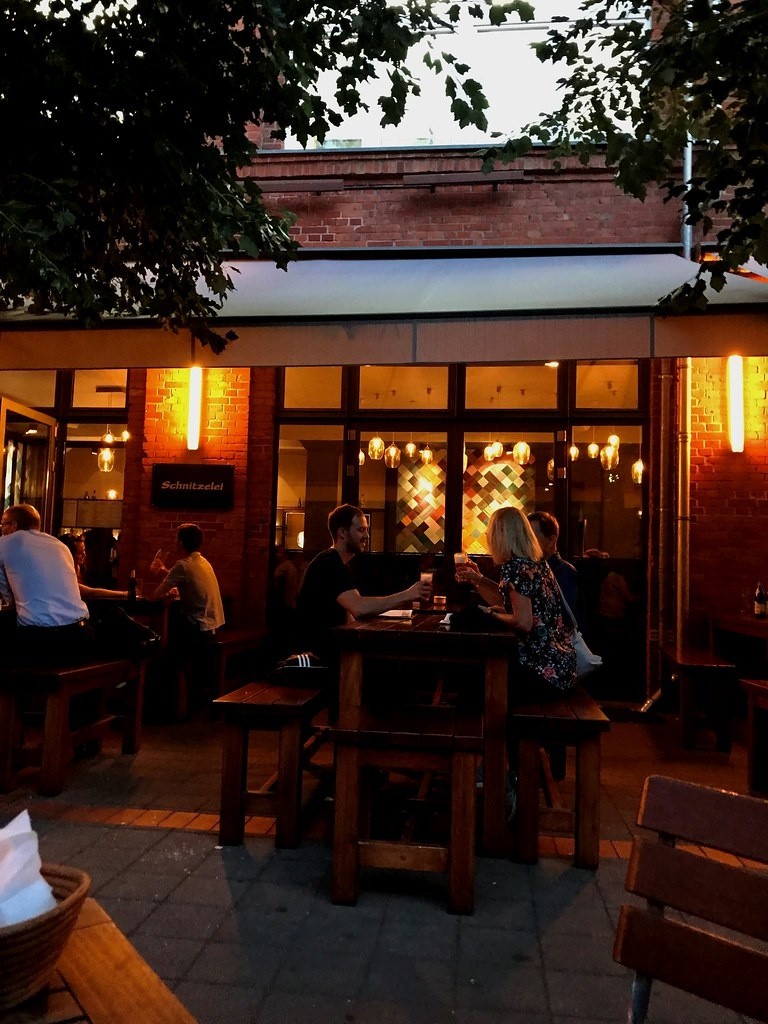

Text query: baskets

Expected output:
[0,864,91,1009]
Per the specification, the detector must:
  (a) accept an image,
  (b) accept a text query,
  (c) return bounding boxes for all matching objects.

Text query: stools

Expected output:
[330,705,485,916]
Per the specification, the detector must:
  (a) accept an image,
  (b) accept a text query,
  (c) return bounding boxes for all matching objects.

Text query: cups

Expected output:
[454,553,468,582]
[420,572,433,601]
[149,549,170,575]
[434,596,446,604]
[172,587,181,600]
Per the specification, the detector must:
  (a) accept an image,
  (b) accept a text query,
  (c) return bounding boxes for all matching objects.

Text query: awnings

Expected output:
[0,254,768,366]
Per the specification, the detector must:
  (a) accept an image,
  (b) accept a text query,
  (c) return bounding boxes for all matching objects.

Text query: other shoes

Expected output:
[73,736,100,759]
[435,765,483,788]
[505,776,518,820]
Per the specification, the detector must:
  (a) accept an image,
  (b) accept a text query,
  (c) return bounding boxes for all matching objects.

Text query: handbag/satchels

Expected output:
[554,579,602,679]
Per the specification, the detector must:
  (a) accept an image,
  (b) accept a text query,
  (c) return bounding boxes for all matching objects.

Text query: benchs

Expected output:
[0,659,145,798]
[510,687,610,869]
[662,644,768,793]
[209,677,333,850]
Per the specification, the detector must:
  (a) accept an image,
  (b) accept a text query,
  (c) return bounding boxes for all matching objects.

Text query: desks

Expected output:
[703,607,768,654]
[330,601,520,859]
[0,594,235,642]
[0,895,199,1024]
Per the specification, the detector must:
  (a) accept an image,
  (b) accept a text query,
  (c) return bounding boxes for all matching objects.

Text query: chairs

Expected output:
[614,776,767,1024]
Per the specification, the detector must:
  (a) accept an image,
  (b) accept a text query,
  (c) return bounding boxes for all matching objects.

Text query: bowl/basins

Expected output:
[0,862,93,1011]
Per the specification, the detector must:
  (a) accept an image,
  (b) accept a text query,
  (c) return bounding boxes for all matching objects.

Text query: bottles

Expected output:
[753,581,766,619]
[360,494,365,508]
[92,489,97,499]
[127,569,137,601]
[84,491,89,499]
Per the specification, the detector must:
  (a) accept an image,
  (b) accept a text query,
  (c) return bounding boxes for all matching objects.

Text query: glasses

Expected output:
[0,520,12,530]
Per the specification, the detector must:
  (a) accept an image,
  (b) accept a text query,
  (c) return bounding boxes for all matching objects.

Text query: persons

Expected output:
[448,507,579,717]
[0,504,92,694]
[596,560,639,663]
[295,503,431,714]
[155,522,225,642]
[56,533,138,601]
[80,528,119,588]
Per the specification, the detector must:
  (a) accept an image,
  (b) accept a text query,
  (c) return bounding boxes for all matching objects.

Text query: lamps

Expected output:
[588,426,599,458]
[368,432,385,460]
[384,432,401,468]
[405,432,416,457]
[97,393,116,472]
[420,432,433,465]
[570,426,579,461]
[358,432,365,465]
[600,426,620,471]
[512,432,530,465]
[631,427,644,483]
[484,432,494,461]
[491,432,503,457]
